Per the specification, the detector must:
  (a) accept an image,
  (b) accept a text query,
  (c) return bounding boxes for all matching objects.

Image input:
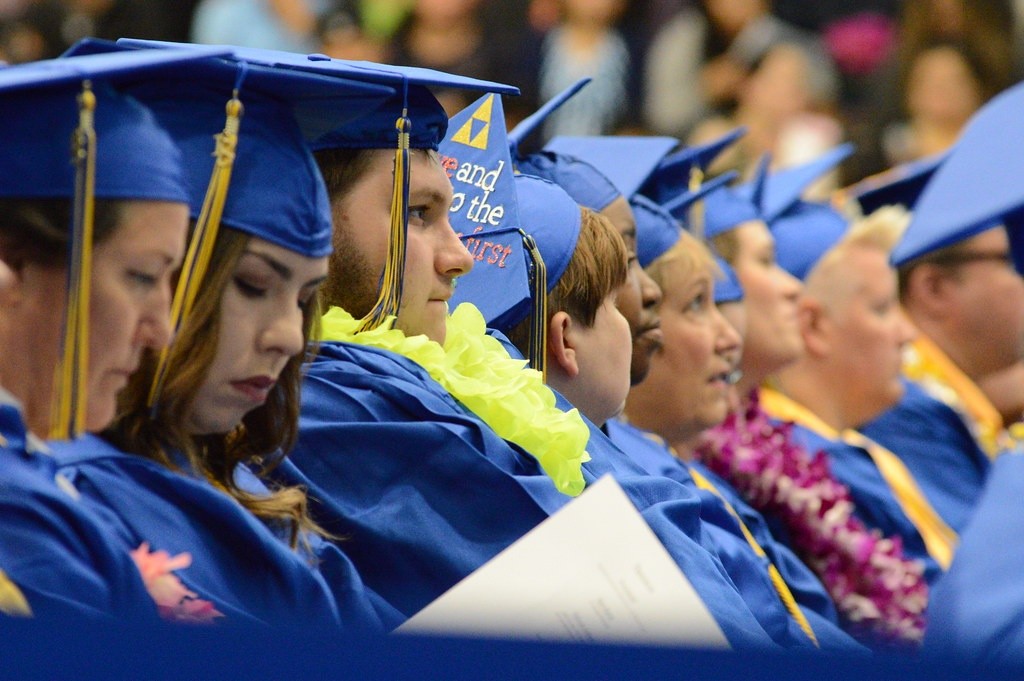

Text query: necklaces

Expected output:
[309,294,590,498]
[693,402,928,649]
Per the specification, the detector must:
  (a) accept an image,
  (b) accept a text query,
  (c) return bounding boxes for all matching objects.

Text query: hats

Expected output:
[118,35,521,334]
[1,44,235,442]
[56,36,397,404]
[889,79,1024,283]
[434,74,954,391]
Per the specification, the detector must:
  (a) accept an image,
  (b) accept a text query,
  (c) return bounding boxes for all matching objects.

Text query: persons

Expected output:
[0,0,1024,681]
